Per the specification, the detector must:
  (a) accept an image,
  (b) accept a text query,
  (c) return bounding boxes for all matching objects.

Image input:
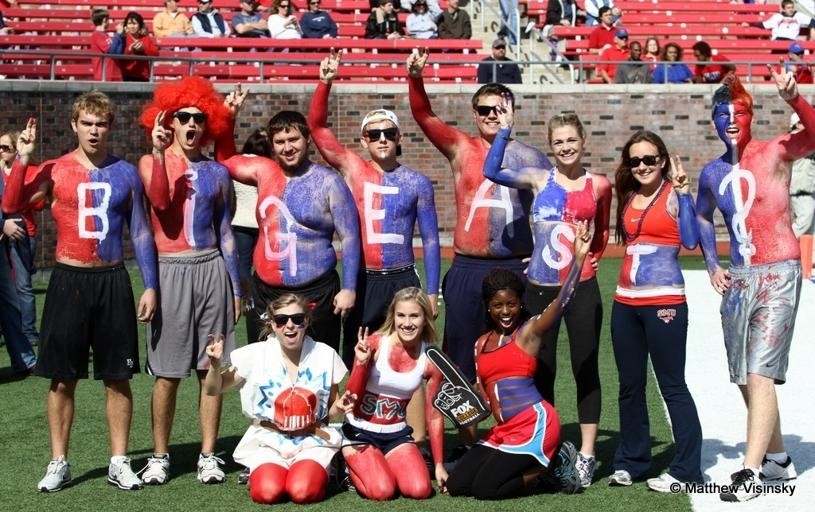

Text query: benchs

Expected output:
[0,0,814,95]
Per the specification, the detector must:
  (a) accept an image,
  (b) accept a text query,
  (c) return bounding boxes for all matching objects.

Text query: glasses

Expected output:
[475,105,497,117]
[272,313,307,326]
[362,128,399,140]
[629,153,660,167]
[175,111,206,125]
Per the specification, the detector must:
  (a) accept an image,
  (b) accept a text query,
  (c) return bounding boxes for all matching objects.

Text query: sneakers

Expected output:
[731,454,797,482]
[107,459,143,490]
[142,455,170,484]
[238,467,251,483]
[37,462,71,493]
[331,455,357,492]
[608,470,633,485]
[554,441,597,494]
[646,473,704,493]
[423,454,435,475]
[444,444,470,474]
[197,453,226,483]
[720,469,765,501]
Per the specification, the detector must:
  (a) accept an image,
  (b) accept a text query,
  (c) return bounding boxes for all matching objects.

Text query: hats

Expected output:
[362,109,401,155]
